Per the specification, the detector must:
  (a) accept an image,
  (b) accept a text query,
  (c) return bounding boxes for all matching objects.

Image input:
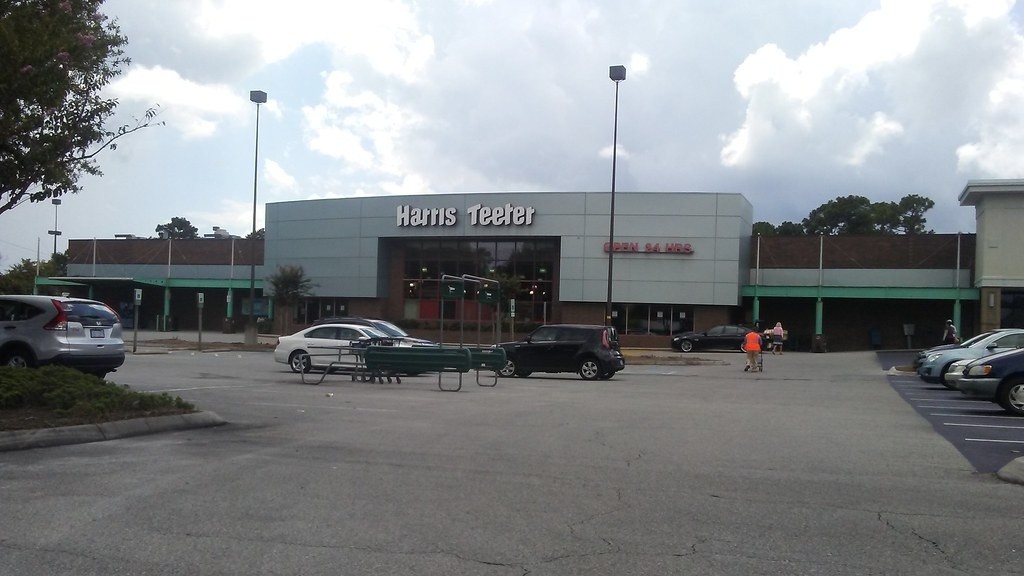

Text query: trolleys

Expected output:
[745,345,763,372]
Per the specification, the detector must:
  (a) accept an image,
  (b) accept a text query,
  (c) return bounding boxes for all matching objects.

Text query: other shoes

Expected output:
[779,352,783,356]
[743,364,750,372]
[772,350,776,354]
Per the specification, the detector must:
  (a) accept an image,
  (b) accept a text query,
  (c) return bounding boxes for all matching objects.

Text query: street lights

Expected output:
[604,64,629,326]
[243,89,270,347]
[52,197,61,254]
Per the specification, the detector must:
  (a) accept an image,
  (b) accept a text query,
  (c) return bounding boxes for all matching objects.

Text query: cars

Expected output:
[958,346,1024,417]
[912,328,1024,371]
[918,330,1024,391]
[671,323,771,353]
[944,359,976,389]
[275,324,410,373]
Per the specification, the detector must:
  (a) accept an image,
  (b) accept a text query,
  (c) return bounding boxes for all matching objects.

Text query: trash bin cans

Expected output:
[157,314,175,332]
[222,316,235,334]
[257,317,272,334]
[812,333,827,353]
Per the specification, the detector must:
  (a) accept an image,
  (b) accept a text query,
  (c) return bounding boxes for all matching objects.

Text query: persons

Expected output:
[744,327,762,372]
[772,322,784,356]
[943,319,959,344]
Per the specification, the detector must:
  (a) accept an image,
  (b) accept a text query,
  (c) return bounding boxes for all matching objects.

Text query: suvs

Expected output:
[0,295,125,380]
[309,316,434,376]
[484,323,625,381]
